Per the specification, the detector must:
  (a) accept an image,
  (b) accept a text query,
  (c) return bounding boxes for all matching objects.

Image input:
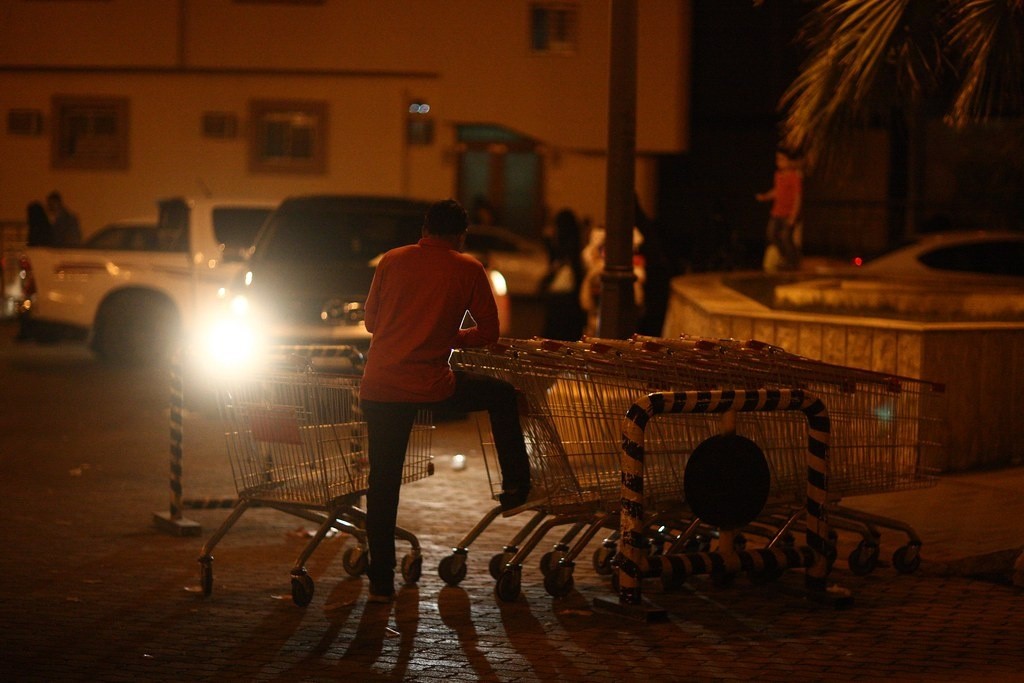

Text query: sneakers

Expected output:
[498,490,546,516]
[368,572,398,602]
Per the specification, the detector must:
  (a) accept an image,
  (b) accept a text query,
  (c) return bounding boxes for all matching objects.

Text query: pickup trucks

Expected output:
[0,191,295,365]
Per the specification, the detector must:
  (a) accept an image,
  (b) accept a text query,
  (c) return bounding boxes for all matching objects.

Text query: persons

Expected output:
[755,142,806,274]
[359,198,563,597]
[45,189,83,249]
[25,200,60,248]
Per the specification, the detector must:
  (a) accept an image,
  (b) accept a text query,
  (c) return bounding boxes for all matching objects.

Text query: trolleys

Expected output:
[203,365,438,608]
[434,333,932,609]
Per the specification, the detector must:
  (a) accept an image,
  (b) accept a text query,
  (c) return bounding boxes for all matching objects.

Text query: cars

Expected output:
[776,229,1024,322]
[466,225,573,299]
[224,193,453,400]
